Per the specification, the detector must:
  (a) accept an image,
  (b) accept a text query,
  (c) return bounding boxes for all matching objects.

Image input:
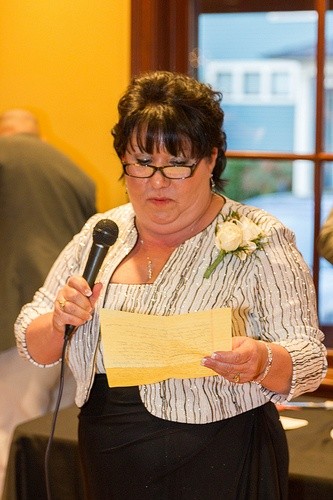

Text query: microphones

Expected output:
[65,219,119,340]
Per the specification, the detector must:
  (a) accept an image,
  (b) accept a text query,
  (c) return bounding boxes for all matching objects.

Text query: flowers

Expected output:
[203,207,270,279]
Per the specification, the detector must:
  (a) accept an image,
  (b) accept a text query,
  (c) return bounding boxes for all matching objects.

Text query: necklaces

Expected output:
[141,240,184,280]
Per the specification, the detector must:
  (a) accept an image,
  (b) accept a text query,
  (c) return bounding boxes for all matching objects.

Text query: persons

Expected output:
[12,72,329,500]
[0,111,97,500]
[315,211,333,266]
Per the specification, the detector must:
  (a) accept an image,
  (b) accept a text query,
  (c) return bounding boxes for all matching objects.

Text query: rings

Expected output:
[61,300,68,311]
[233,373,240,384]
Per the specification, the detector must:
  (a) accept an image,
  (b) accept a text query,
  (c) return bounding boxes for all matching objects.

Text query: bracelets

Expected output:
[251,341,273,385]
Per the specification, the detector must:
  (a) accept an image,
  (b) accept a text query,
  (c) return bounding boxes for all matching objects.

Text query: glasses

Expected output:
[120,152,204,179]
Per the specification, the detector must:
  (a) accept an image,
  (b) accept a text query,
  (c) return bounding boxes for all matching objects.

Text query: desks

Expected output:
[0,401,333,500]
